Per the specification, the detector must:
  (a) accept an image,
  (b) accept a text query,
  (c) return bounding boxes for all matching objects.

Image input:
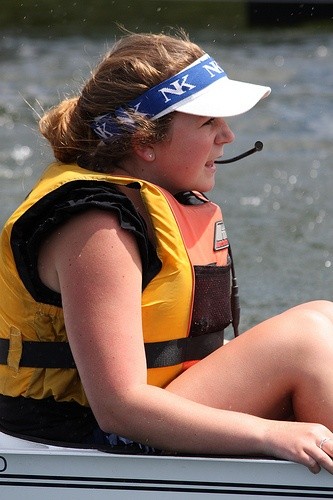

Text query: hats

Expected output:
[91,53,271,138]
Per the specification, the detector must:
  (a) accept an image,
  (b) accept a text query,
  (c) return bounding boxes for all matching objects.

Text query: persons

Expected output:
[1,35,333,475]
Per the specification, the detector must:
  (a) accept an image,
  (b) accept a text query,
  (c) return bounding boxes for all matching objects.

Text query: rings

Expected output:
[319,437,331,449]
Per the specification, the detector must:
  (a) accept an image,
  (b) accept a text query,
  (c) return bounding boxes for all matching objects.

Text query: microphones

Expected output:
[214,140,263,164]
